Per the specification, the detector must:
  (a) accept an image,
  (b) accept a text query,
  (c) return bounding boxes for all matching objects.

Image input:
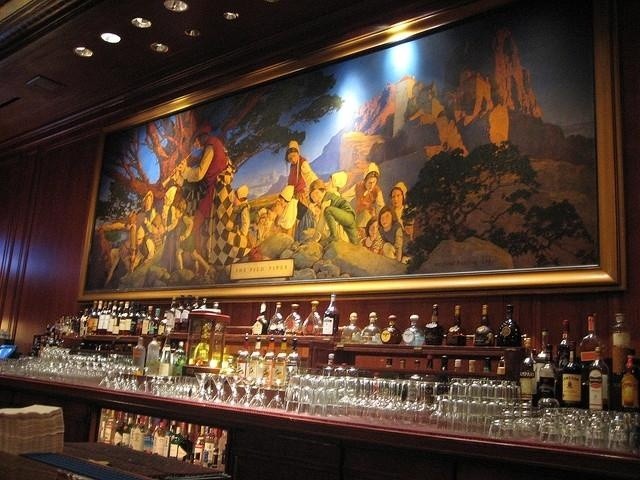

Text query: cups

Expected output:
[10,346,227,406]
[227,361,640,458]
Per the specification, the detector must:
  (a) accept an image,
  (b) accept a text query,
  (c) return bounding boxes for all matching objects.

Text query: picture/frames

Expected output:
[75,1,633,304]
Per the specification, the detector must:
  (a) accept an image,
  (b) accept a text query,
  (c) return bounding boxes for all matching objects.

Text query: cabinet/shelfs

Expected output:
[0,334,639,479]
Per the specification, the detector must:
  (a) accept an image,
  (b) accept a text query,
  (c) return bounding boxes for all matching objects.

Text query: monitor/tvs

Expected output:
[0,344,17,359]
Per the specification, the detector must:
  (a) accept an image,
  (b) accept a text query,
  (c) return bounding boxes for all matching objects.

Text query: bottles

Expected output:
[48,294,221,338]
[246,295,640,412]
[96,407,228,473]
